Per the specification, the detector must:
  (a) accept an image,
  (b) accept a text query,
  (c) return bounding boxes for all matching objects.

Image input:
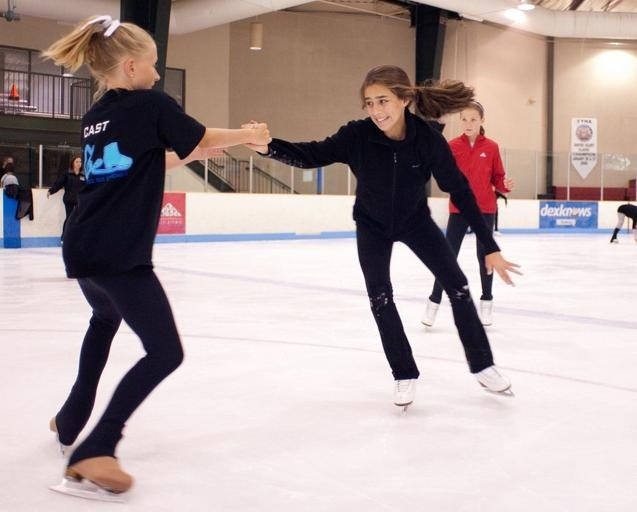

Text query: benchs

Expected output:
[0,93,36,110]
[537,179,636,201]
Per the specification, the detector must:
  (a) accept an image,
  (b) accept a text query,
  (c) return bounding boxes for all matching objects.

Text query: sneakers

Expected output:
[480,299,493,327]
[50,417,132,493]
[421,299,439,326]
[477,365,510,392]
[394,379,414,405]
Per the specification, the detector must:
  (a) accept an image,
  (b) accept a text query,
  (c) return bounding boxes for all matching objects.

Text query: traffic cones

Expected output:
[7,84,19,100]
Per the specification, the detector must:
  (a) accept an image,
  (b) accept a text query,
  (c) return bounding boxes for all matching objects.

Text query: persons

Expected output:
[421,101,512,328]
[40,16,274,494]
[0,165,19,188]
[243,65,514,406]
[46,156,88,244]
[0,157,13,182]
[610,204,637,243]
